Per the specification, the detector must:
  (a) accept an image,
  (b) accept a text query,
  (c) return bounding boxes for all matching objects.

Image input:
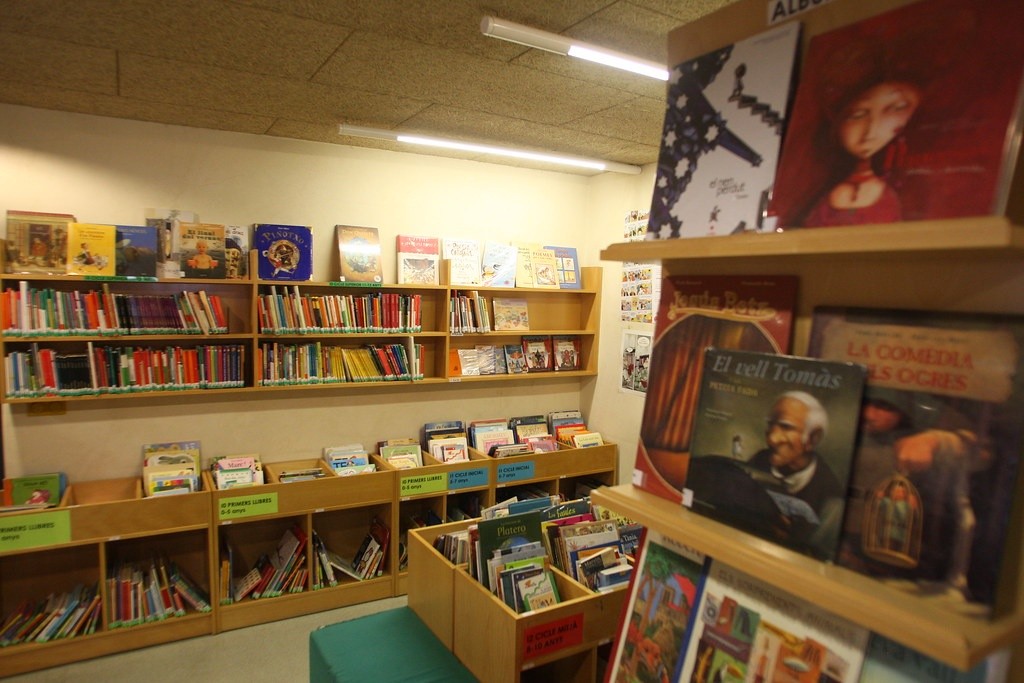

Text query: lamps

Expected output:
[336,124,645,175]
[477,14,670,81]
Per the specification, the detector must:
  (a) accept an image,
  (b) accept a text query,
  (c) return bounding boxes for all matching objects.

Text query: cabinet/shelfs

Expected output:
[0,236,604,405]
[600,0,1024,683]
[0,440,629,679]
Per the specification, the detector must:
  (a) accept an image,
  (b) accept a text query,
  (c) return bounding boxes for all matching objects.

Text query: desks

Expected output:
[309,605,480,683]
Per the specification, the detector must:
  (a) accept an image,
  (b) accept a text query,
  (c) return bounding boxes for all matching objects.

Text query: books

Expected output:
[634,0,1024,241]
[604,526,1009,683]
[644,272,1024,622]
[434,495,642,615]
[0,210,580,400]
[0,409,603,648]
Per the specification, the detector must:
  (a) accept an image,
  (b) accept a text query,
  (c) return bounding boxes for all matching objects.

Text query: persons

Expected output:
[865,387,1001,587]
